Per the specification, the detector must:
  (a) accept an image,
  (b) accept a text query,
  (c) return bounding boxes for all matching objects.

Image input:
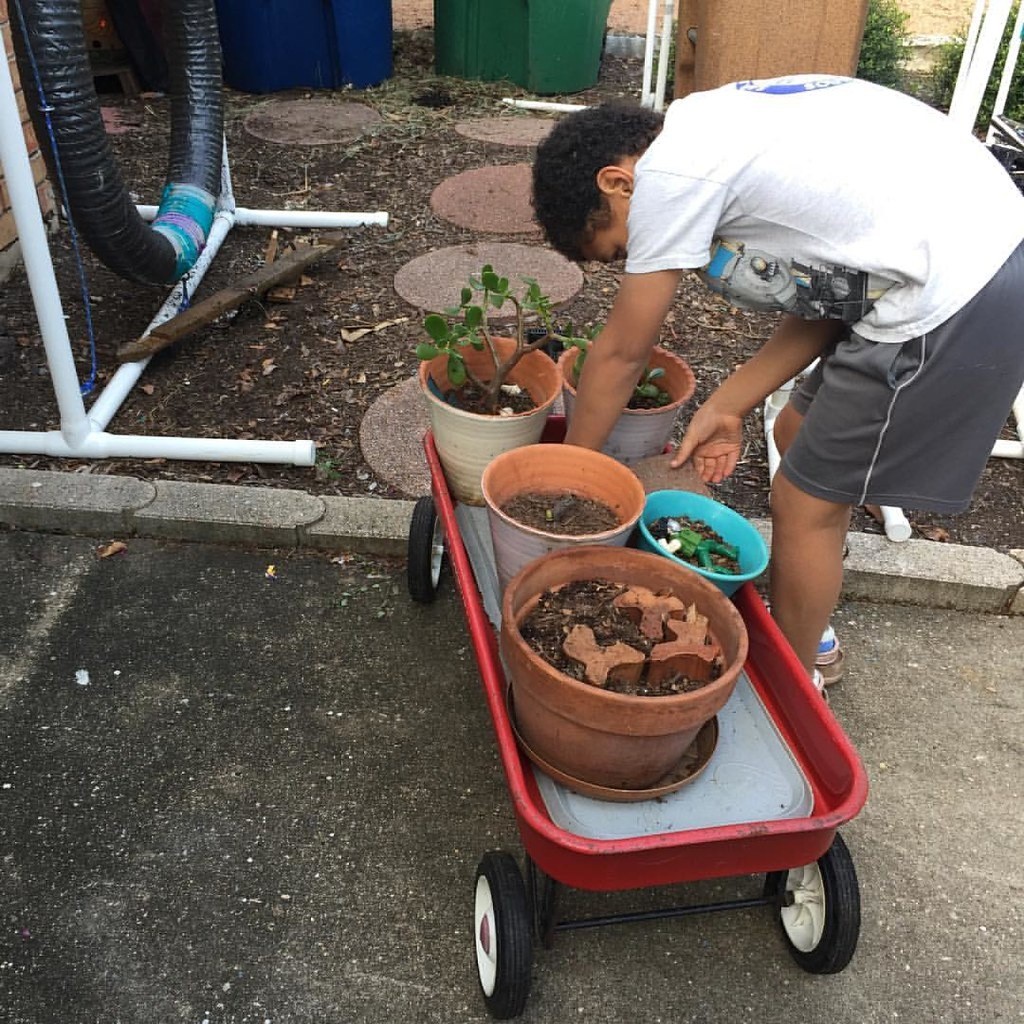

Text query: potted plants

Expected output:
[413,263,604,512]
[557,339,696,461]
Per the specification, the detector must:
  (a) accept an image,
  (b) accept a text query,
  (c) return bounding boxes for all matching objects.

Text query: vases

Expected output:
[478,442,647,594]
[498,545,750,803]
[638,490,771,601]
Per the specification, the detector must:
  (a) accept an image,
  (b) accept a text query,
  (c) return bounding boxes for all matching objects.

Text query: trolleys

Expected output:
[407,326,871,1020]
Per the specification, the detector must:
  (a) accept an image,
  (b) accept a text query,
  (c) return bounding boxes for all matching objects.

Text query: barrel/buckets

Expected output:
[641,489,769,599]
[98,1,612,92]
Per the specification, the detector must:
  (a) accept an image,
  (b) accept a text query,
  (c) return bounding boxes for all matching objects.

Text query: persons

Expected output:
[532,73,1022,708]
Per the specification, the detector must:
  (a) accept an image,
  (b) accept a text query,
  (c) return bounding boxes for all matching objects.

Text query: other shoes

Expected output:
[814,670,829,705]
[815,636,845,684]
